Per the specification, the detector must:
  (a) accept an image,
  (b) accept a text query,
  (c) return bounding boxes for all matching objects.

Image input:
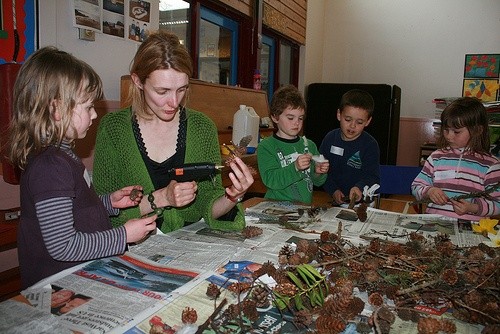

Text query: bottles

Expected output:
[254,70,261,90]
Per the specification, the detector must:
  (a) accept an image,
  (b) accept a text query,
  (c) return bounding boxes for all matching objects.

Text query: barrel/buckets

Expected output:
[232,105,260,148]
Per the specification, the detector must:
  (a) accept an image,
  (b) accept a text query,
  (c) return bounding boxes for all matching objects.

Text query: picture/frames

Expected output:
[462,78,498,101]
[464,53,500,78]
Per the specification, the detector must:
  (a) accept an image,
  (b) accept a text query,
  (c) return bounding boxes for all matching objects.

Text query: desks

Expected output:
[0,197,500,334]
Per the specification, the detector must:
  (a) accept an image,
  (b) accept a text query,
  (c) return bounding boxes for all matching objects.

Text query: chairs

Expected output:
[376,164,423,214]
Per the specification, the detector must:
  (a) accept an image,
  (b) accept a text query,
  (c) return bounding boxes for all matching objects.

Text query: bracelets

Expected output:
[148,191,171,216]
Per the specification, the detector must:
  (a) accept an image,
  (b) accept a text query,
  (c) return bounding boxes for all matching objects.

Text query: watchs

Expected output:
[225,185,245,203]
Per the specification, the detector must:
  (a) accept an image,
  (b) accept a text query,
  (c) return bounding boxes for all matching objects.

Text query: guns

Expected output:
[166,161,225,183]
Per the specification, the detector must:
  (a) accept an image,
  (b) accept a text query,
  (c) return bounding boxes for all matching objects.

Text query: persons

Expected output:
[319,89,381,207]
[257,84,329,204]
[51,289,94,316]
[411,96,500,220]
[93,31,254,234]
[0,45,158,288]
